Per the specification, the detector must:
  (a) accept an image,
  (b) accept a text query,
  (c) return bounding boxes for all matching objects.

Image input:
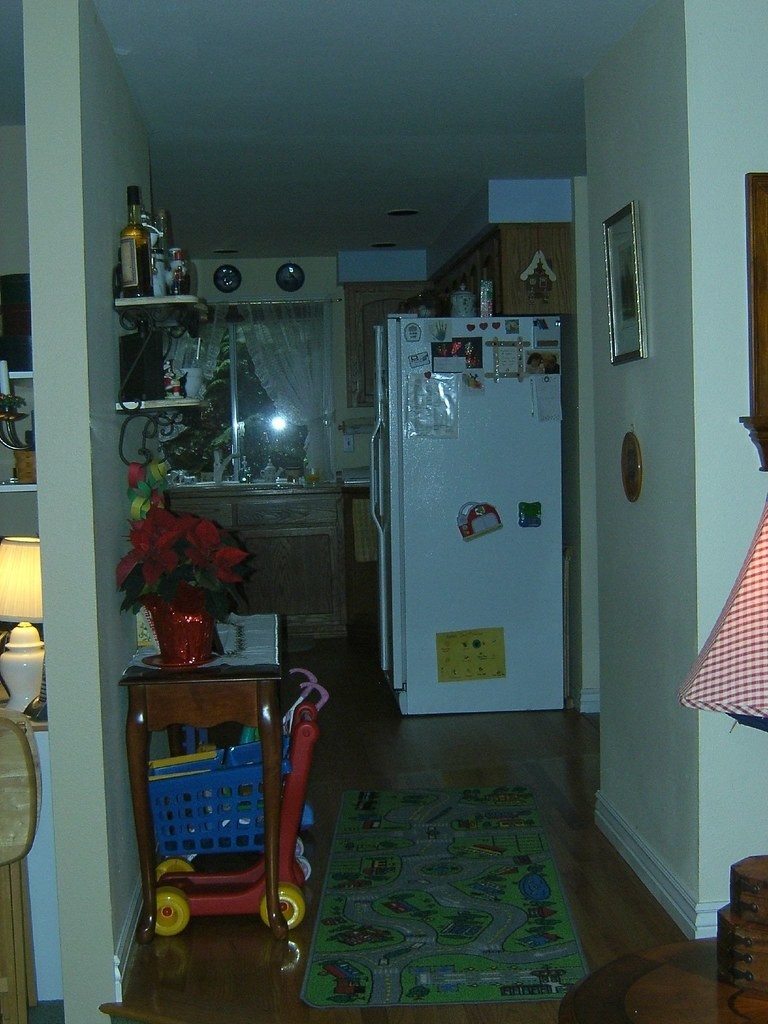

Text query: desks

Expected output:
[118,613,288,943]
[560,938,768,1024]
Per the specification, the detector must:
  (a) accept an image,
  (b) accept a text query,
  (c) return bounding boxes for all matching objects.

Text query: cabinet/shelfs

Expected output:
[344,281,427,408]
[166,494,347,638]
[429,223,573,315]
[0,372,40,492]
[113,294,200,468]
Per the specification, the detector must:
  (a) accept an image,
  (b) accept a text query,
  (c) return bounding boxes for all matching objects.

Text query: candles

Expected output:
[0,360,10,396]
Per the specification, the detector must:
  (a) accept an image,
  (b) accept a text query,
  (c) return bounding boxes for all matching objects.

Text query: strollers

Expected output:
[189,668,329,838]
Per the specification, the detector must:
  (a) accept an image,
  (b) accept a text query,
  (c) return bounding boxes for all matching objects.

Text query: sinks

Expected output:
[204,486,290,492]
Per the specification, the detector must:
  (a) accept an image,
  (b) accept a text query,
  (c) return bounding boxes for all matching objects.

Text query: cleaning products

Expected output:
[247,467,251,472]
[239,455,250,483]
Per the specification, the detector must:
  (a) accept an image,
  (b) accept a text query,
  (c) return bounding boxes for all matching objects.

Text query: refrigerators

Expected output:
[370,313,564,716]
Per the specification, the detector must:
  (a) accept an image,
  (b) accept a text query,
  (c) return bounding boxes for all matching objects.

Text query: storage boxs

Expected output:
[716,855,768,993]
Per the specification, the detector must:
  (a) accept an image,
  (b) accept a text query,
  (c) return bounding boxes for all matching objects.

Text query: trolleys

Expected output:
[144,704,320,936]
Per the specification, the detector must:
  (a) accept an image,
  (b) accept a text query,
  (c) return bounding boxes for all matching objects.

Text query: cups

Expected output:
[285,467,300,481]
[304,467,320,484]
[181,368,202,398]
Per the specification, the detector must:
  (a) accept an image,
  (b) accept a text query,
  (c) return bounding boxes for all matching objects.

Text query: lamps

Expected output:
[1,537,45,714]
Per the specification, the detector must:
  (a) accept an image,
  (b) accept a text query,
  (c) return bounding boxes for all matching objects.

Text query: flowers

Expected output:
[111,502,249,625]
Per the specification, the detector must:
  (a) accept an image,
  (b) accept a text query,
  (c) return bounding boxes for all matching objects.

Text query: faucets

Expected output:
[246,473,253,484]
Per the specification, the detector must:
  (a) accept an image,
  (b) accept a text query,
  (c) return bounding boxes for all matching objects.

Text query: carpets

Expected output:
[299,784,589,1009]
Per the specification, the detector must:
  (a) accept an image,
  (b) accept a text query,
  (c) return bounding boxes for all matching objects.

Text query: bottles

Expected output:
[121,185,190,299]
[449,283,477,317]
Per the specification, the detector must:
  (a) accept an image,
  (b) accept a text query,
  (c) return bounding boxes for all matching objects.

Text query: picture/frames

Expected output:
[603,200,649,364]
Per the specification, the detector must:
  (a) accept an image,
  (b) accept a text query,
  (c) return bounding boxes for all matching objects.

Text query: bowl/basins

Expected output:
[407,289,441,318]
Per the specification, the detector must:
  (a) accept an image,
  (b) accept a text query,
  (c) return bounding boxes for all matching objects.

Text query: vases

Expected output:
[138,583,220,668]
